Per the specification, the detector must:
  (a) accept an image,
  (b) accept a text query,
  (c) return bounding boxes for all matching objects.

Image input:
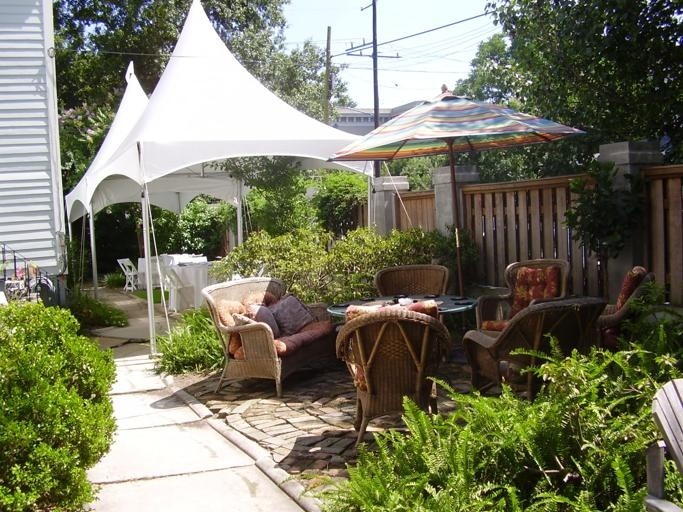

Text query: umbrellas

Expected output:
[325,85,589,299]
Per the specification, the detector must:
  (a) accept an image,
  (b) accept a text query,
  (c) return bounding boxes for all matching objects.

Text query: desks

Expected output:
[160,253,207,261]
[169,262,214,309]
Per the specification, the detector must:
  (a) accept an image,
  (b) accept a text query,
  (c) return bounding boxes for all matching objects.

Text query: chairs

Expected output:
[116,254,192,314]
